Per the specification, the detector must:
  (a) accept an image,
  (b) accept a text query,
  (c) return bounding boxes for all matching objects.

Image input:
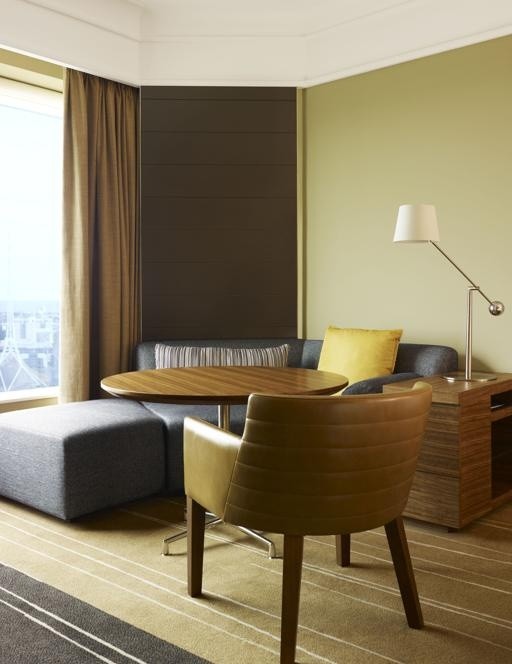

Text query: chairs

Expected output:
[183,380,434,663]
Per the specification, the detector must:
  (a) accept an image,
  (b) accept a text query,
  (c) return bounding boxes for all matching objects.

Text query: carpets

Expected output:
[0,561,214,664]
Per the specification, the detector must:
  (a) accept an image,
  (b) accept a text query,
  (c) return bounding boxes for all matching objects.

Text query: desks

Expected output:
[101,365,349,558]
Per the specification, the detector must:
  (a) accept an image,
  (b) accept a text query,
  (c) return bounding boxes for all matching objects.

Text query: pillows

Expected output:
[317,324,403,395]
[154,341,290,368]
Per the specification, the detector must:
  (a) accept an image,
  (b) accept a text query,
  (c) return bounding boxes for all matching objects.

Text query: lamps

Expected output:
[393,201,505,383]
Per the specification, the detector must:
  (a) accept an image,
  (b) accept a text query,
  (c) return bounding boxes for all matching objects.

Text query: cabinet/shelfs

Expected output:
[380,369,511,533]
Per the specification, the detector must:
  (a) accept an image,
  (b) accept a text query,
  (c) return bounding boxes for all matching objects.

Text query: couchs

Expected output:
[1,339,457,522]
